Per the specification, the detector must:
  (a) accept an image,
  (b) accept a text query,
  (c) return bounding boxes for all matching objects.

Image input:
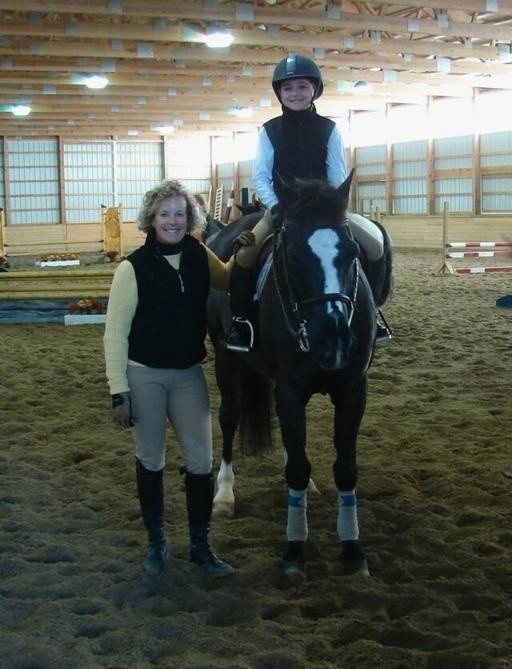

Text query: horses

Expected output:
[212,168,377,580]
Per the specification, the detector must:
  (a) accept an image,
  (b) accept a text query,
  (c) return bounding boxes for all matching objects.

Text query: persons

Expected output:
[225,53,391,352]
[193,194,228,244]
[104,181,255,577]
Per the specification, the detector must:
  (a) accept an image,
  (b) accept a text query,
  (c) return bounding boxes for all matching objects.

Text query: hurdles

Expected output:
[430,240,512,276]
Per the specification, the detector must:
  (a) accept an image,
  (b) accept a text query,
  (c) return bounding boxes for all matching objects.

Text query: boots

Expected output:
[364,248,388,338]
[135,455,167,578]
[227,253,262,344]
[179,465,236,579]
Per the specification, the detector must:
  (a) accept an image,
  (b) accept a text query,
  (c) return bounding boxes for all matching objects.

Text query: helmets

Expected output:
[272,54,323,99]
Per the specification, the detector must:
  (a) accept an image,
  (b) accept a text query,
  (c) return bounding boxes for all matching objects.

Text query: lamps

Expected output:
[202,19,236,49]
